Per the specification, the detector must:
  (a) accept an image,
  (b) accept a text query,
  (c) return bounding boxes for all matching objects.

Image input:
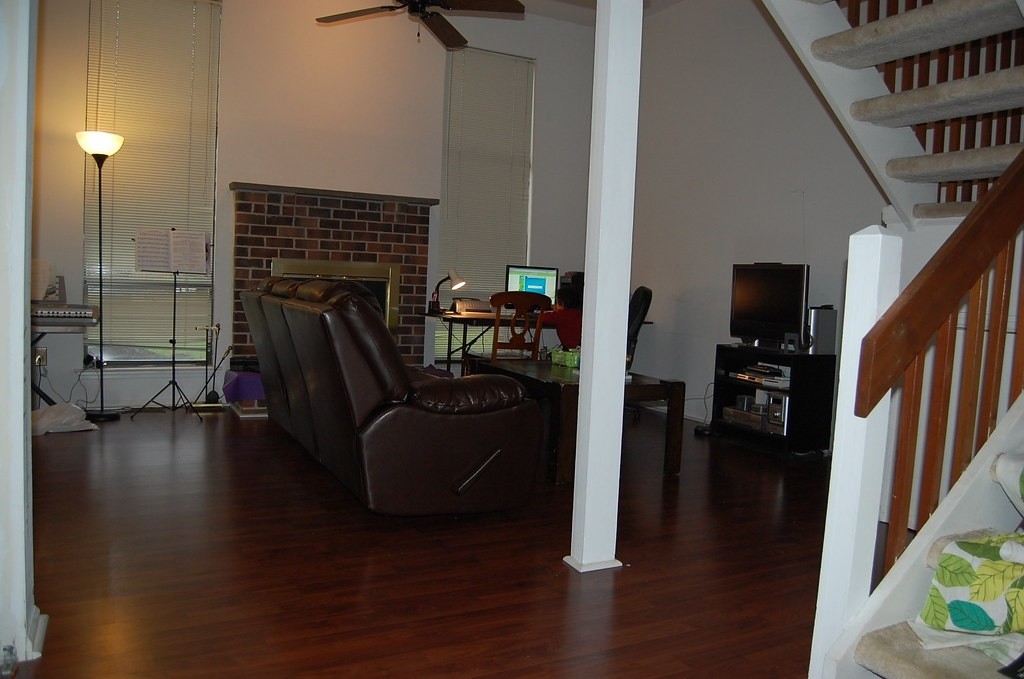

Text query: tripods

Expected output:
[130,231,207,422]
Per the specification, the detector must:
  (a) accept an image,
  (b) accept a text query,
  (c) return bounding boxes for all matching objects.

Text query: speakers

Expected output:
[809,309,837,355]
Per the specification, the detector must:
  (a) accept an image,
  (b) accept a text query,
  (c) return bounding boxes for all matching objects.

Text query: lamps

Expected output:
[429,270,466,314]
[76,131,125,424]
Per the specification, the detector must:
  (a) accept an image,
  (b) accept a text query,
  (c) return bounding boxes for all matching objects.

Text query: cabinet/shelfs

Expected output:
[711,342,836,463]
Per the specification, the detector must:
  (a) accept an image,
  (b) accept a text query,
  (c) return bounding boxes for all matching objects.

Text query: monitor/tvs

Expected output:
[505,265,558,311]
[730,264,810,351]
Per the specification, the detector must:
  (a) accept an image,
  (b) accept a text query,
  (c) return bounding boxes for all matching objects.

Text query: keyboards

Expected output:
[461,300,491,311]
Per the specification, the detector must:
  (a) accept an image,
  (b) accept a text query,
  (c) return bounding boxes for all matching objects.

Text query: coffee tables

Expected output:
[471,357,686,485]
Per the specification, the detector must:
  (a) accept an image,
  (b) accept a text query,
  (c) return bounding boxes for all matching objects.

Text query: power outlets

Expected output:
[35,347,47,366]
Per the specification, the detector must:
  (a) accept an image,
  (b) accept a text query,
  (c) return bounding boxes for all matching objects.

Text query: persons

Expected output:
[542,287,583,352]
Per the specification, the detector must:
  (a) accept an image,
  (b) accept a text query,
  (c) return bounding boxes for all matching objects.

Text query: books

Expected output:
[135,228,207,275]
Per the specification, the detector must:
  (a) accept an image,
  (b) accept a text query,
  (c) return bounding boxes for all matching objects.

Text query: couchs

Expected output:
[240,276,548,516]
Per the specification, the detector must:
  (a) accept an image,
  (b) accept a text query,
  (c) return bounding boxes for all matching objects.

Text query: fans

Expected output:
[315,0,525,49]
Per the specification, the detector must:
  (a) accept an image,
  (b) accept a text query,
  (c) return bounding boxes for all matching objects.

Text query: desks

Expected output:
[422,312,554,377]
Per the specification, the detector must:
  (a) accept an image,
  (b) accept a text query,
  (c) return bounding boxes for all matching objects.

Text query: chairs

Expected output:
[463,291,552,377]
[625,286,652,369]
